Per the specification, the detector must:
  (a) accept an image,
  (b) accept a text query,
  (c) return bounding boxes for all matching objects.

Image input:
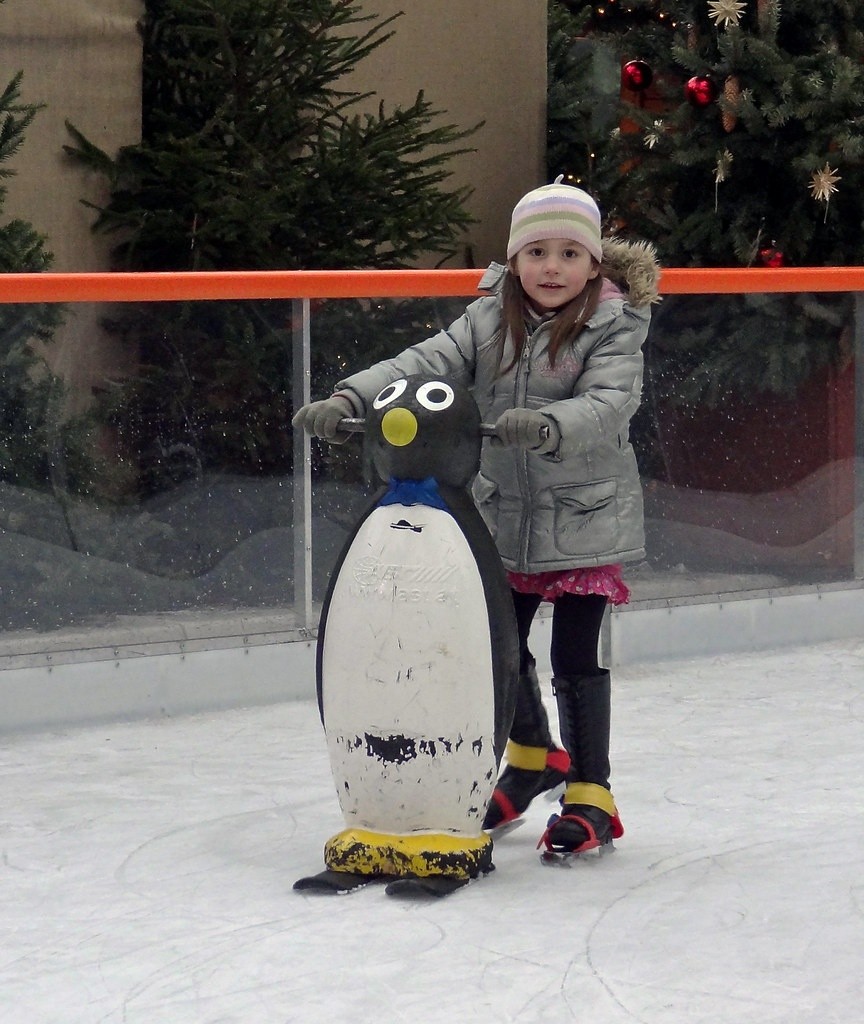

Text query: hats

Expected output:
[507,174,603,263]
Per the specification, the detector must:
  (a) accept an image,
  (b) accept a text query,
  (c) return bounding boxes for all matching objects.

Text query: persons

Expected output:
[292,173,663,866]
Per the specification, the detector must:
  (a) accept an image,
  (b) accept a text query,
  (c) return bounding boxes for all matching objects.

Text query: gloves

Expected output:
[291,397,356,446]
[489,408,555,454]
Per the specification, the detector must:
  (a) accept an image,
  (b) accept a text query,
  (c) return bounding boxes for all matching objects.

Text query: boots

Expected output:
[483,659,573,828]
[539,665,617,848]
